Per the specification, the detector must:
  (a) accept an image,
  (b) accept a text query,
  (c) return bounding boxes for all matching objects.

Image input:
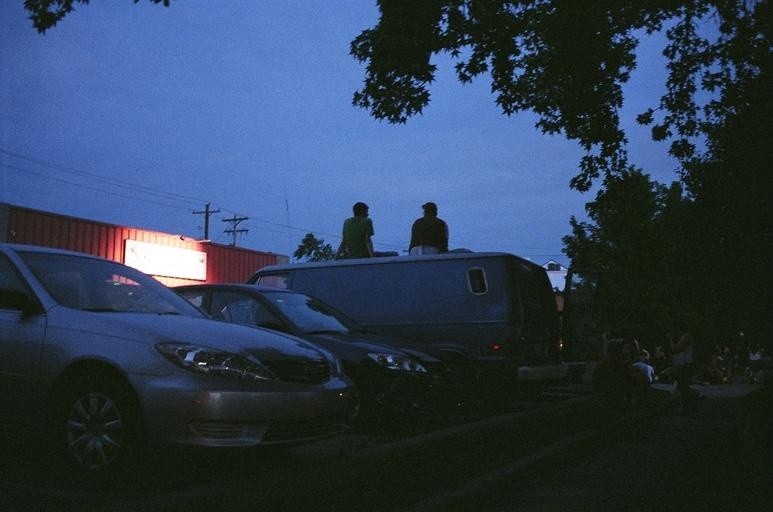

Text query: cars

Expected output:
[166,283,462,438]
[0,244,357,492]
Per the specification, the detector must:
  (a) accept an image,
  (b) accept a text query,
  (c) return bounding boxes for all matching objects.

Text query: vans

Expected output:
[239,253,559,409]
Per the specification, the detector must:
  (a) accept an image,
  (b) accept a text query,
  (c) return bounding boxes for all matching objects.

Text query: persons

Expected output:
[333,201,400,257]
[406,201,450,253]
[634,311,771,402]
